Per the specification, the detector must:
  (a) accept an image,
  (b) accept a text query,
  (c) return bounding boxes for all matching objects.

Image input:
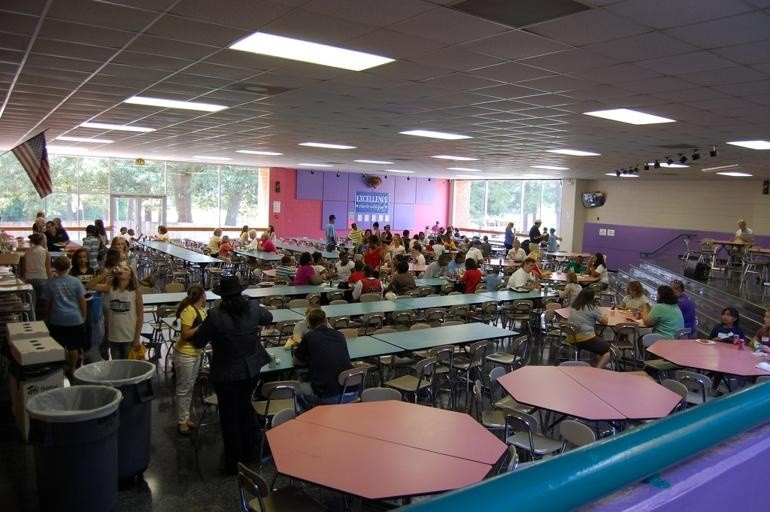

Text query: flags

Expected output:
[10,132,53,198]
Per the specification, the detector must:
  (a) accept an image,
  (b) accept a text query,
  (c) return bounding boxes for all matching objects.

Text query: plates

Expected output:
[696,338,716,345]
[53,242,65,247]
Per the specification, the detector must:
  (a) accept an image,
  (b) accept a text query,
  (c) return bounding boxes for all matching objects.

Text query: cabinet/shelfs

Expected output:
[0,269,39,333]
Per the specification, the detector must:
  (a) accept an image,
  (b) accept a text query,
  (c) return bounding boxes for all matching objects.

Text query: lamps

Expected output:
[677,153,688,164]
[664,157,674,166]
[634,165,640,172]
[692,148,701,160]
[644,163,651,171]
[710,144,719,157]
[628,168,634,174]
[616,170,620,179]
[622,170,628,174]
[655,162,660,168]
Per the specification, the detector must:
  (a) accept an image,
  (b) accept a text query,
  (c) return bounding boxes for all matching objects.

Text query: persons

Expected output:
[741,310,770,390]
[19,211,171,373]
[174,285,208,435]
[544,272,582,328]
[526,221,548,263]
[507,258,542,335]
[565,256,585,276]
[527,252,553,280]
[732,218,753,273]
[208,225,276,263]
[294,307,335,381]
[504,223,515,259]
[708,307,746,395]
[636,286,685,367]
[568,287,611,369]
[277,208,503,301]
[292,309,360,417]
[578,252,610,304]
[192,274,272,477]
[614,281,650,350]
[548,228,558,268]
[540,227,549,248]
[505,239,526,261]
[671,279,698,340]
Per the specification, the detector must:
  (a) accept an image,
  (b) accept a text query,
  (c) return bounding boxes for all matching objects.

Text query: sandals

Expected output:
[188,420,197,429]
[177,423,190,433]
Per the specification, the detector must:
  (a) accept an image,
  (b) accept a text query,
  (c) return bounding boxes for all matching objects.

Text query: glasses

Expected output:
[366,241,373,243]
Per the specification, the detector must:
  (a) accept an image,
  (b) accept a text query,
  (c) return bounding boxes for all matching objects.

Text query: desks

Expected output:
[706,240,755,270]
[120,221,607,327]
[207,335,407,373]
[371,321,520,357]
[496,364,683,422]
[265,399,509,500]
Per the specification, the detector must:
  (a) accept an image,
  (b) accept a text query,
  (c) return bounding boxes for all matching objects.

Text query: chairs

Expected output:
[236,461,324,510]
[485,334,527,373]
[674,370,713,404]
[489,367,536,412]
[728,242,746,277]
[607,323,640,371]
[642,333,684,380]
[561,418,596,453]
[269,321,298,348]
[410,343,455,384]
[703,241,720,271]
[328,365,362,402]
[557,323,584,362]
[450,340,488,402]
[383,356,437,410]
[558,361,591,366]
[661,380,689,412]
[473,380,522,432]
[361,387,401,400]
[683,239,704,266]
[261,379,303,422]
[501,408,563,463]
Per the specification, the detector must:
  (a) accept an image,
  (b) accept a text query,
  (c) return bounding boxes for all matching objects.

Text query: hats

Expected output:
[535,220,541,223]
[212,276,249,296]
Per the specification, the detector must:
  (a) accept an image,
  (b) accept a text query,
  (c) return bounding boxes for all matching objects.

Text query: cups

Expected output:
[636,311,640,320]
[732,333,739,345]
[738,339,745,351]
[149,236,151,241]
[761,336,770,346]
[143,237,145,242]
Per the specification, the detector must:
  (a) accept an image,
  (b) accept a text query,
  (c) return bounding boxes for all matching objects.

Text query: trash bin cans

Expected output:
[73,358,156,479]
[26,385,120,511]
